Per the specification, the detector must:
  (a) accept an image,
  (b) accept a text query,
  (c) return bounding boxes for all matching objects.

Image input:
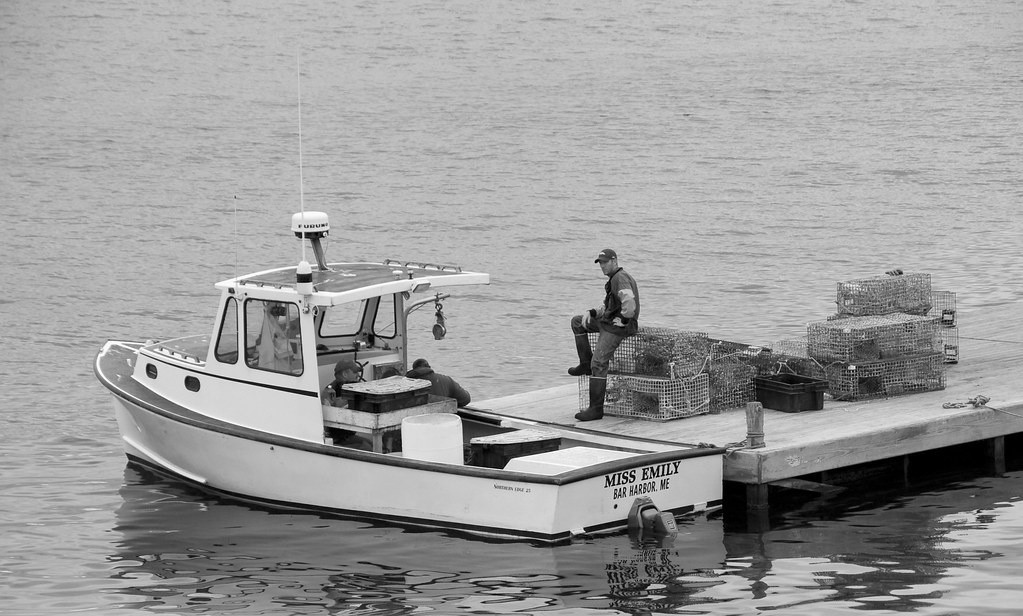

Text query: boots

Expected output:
[575,377,606,421]
[568,333,593,376]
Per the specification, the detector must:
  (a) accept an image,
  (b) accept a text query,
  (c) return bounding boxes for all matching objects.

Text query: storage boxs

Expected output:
[341,375,433,413]
[576,272,958,420]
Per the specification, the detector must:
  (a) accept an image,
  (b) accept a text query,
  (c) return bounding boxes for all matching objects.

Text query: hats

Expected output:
[594,249,617,264]
[334,360,362,374]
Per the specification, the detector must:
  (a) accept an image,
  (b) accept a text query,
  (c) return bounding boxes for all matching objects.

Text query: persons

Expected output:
[320,359,373,451]
[568,249,640,421]
[406,359,470,408]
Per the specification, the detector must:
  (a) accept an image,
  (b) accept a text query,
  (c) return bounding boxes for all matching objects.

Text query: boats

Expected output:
[92,47,727,547]
